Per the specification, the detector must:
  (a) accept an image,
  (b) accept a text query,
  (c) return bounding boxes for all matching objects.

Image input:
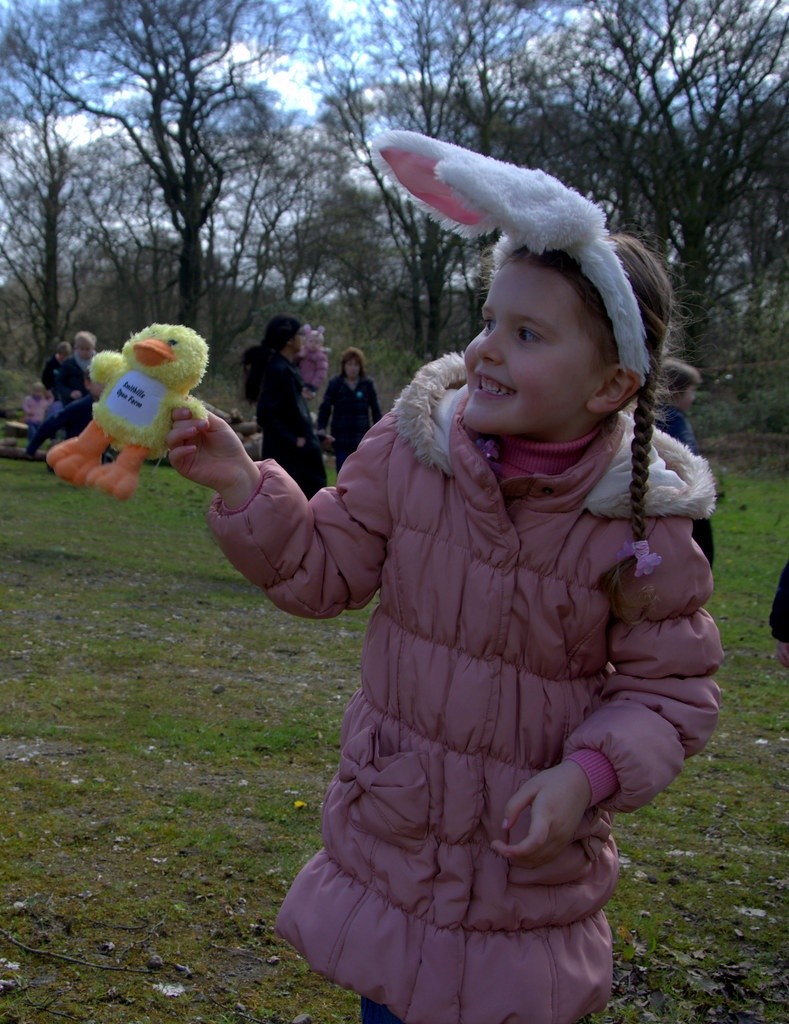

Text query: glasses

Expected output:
[298,329,307,337]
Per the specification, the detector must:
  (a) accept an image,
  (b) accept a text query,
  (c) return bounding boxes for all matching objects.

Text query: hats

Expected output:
[304,324,324,342]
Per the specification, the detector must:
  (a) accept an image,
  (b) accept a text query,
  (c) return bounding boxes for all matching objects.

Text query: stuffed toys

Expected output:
[47,322,209,503]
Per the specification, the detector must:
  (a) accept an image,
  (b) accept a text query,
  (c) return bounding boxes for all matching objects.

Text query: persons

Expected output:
[241,314,383,502]
[650,359,715,574]
[169,130,724,1024]
[769,560,789,669]
[22,332,112,471]
